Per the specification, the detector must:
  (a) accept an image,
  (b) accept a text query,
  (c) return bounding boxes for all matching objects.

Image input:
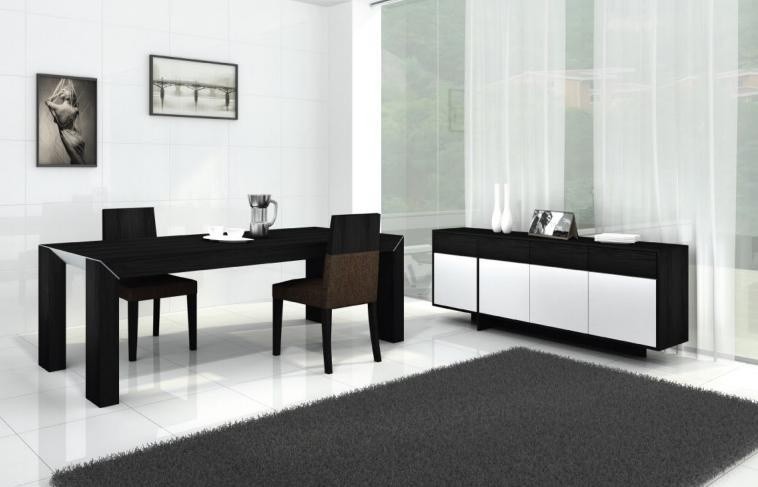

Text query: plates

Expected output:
[203,235,254,243]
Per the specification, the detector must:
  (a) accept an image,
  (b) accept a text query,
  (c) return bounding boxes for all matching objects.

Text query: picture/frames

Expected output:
[36,72,97,169]
[149,54,239,122]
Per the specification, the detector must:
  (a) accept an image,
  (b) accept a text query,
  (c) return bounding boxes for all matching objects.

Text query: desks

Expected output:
[37,227,405,408]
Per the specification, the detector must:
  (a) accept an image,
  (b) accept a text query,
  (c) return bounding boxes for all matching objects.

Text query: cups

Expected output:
[208,226,245,239]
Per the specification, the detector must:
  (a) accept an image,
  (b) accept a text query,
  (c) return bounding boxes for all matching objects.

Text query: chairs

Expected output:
[273,213,382,375]
[101,208,197,361]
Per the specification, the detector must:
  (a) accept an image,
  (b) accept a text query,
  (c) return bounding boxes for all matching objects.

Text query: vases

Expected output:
[491,184,513,234]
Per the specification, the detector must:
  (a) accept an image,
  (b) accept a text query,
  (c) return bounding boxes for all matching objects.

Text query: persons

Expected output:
[43,77,86,164]
[531,211,552,234]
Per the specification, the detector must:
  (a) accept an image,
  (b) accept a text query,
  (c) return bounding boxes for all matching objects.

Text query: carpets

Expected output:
[48,347,758,487]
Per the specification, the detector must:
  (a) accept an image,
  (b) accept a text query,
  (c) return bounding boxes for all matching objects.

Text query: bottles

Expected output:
[492,183,512,234]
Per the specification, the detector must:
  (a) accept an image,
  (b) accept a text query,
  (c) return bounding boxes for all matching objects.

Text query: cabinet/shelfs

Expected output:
[432,227,688,360]
